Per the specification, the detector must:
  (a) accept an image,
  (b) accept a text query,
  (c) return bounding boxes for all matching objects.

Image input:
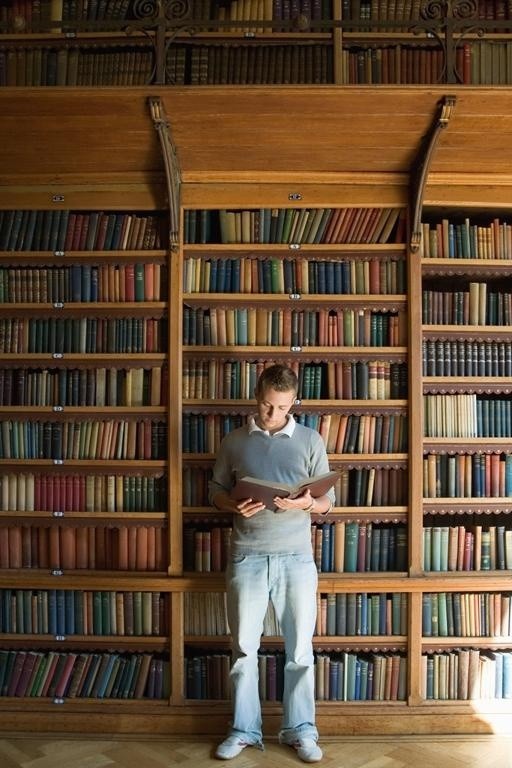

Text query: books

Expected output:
[184,590,407,702]
[0,208,169,700]
[0,0,512,90]
[182,208,411,574]
[420,592,511,701]
[418,218,511,571]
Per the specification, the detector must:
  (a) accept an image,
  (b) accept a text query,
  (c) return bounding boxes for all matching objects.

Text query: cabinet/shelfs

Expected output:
[2,3,512,89]
[3,211,510,738]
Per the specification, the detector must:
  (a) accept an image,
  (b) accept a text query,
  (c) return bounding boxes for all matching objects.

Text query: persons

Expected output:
[206,361,340,763]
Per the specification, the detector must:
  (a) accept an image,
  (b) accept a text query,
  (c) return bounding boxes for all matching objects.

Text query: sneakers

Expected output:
[292,732,323,762]
[214,733,249,760]
